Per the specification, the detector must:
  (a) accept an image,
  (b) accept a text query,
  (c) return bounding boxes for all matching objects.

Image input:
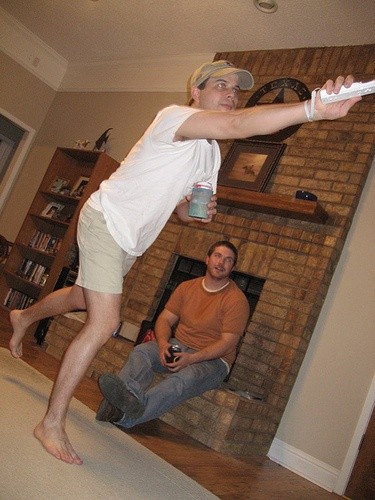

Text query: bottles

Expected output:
[188,179,213,221]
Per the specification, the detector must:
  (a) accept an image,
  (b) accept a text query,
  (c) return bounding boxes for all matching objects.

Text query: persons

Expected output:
[9,61,362,465]
[96,241,250,429]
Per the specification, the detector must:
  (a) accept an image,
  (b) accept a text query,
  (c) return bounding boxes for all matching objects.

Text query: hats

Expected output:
[191,59,254,90]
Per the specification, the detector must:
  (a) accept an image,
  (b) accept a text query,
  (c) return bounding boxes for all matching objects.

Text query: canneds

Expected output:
[165,343,181,366]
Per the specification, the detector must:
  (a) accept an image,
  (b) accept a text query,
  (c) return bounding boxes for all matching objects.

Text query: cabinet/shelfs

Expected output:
[0,147,120,345]
[32,265,78,346]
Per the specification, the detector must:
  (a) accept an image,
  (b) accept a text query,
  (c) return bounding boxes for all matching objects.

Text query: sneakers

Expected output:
[96,399,124,423]
[99,373,145,418]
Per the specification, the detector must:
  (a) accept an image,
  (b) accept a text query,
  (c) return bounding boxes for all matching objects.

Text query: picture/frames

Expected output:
[216,137,288,193]
[40,200,65,219]
[68,176,90,198]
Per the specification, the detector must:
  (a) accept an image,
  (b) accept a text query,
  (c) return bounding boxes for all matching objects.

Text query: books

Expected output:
[4,288,37,310]
[16,259,50,287]
[29,230,63,255]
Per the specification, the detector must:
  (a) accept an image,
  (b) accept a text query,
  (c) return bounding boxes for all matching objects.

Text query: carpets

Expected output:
[1,346,225,500]
